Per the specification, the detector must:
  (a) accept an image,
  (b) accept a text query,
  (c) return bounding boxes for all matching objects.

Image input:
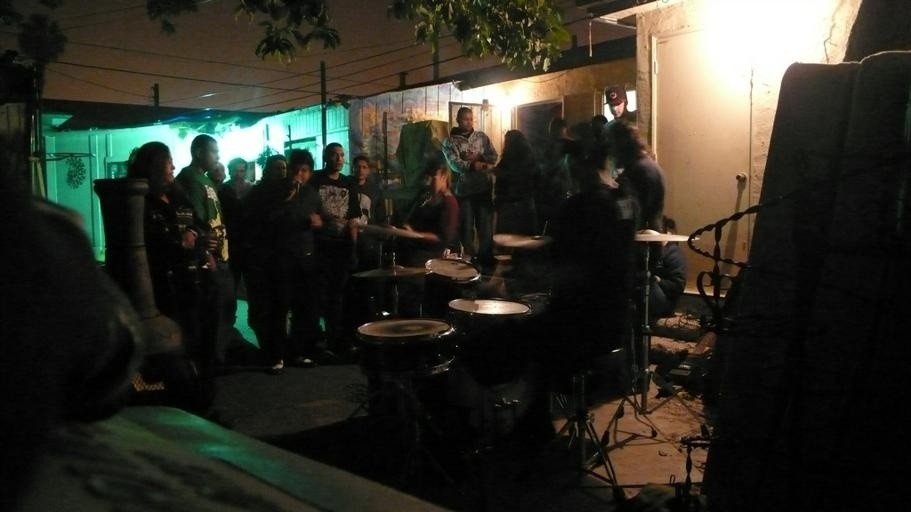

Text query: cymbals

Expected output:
[360,224,437,243]
[632,231,700,242]
[355,269,428,278]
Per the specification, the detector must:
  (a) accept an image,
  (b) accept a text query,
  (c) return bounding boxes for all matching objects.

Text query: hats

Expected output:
[604,88,627,103]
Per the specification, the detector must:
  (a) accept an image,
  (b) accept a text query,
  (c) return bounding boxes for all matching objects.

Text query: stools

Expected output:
[528,344,632,506]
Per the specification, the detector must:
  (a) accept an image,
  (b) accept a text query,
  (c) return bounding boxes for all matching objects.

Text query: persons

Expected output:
[133,86,687,374]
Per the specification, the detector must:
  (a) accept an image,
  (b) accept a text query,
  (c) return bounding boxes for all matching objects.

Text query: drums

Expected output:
[357,319,459,375]
[448,300,532,385]
[427,259,481,298]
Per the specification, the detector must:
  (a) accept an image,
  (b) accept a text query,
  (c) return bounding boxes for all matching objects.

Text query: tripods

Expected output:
[540,334,707,501]
